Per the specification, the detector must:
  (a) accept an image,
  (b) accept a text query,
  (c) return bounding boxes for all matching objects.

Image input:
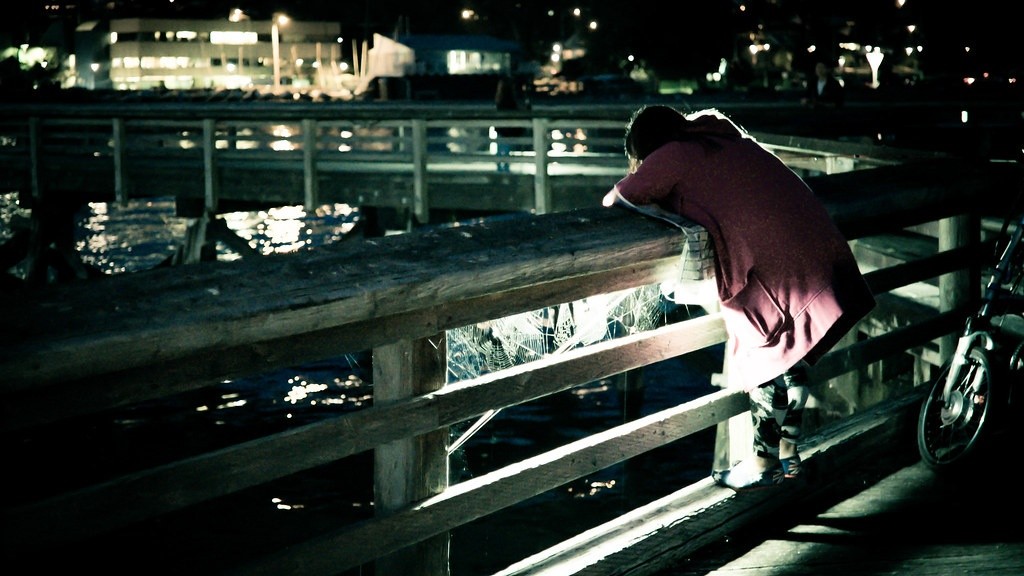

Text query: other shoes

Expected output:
[732,454,803,478]
[712,460,784,491]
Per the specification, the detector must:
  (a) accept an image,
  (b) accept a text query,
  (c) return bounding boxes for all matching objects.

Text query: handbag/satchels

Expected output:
[613,184,718,305]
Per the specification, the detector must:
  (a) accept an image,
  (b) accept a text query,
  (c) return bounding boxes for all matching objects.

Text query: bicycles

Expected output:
[916,221,1024,469]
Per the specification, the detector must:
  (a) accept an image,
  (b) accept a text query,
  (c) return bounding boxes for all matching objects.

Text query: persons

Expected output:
[601,106,876,488]
[799,60,839,141]
